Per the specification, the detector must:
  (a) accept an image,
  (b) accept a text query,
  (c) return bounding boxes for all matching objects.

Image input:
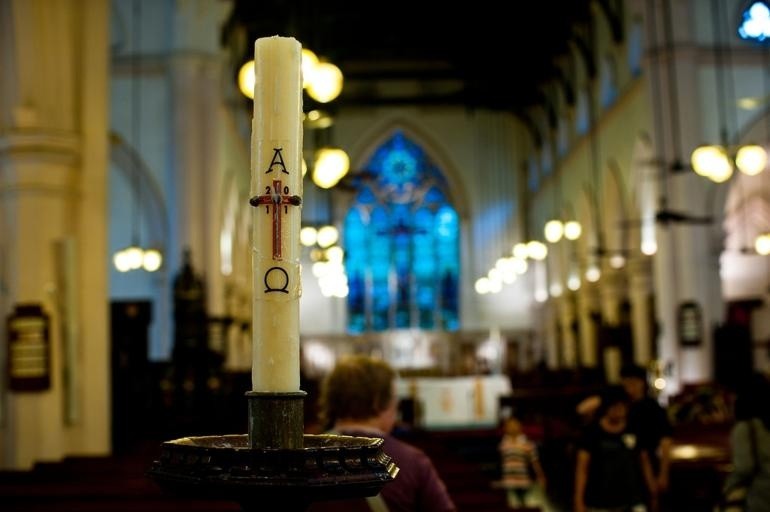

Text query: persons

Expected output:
[573,384,659,512]
[718,393,770,512]
[316,357,457,511]
[618,364,673,512]
[489,417,546,511]
[391,398,443,472]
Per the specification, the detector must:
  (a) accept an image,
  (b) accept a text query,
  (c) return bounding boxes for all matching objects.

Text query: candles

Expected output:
[251,35,303,393]
